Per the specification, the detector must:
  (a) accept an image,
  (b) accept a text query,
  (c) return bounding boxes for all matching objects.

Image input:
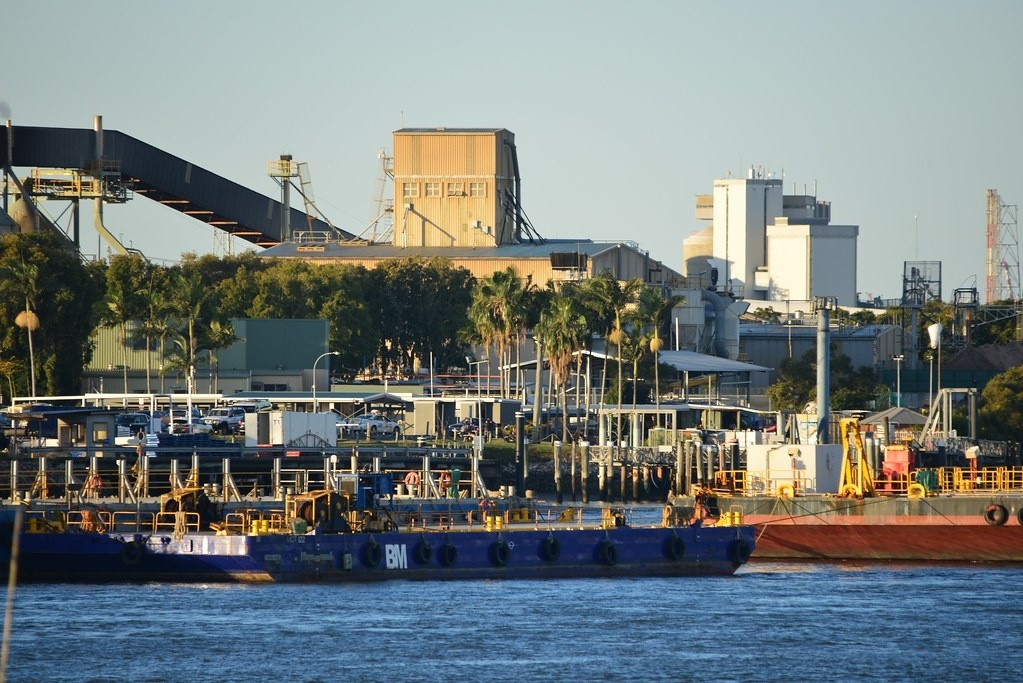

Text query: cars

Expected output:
[116,406,215,436]
[449,418,503,438]
[348,413,401,436]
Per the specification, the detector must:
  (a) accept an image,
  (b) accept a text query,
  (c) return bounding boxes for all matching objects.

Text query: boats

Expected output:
[26,464,758,584]
[686,488,1023,565]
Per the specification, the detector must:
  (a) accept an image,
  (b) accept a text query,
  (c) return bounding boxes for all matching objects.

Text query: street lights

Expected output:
[890,354,905,408]
[313,350,340,413]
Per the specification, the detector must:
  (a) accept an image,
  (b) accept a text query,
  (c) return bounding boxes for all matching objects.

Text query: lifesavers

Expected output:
[600,542,618,566]
[1017,507,1023,526]
[544,538,561,560]
[365,544,381,567]
[122,541,144,568]
[137,432,145,442]
[667,537,685,560]
[492,541,510,567]
[404,472,421,488]
[440,472,452,488]
[731,540,749,564]
[441,544,457,566]
[985,505,1006,527]
[906,484,926,499]
[416,542,432,564]
[480,500,493,513]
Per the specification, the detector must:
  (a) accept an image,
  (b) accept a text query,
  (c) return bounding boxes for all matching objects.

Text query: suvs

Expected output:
[205,407,247,434]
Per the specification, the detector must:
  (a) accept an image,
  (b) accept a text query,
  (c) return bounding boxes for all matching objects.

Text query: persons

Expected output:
[469,423,477,436]
[484,419,495,443]
[578,434,585,444]
[461,418,469,442]
[137,429,146,443]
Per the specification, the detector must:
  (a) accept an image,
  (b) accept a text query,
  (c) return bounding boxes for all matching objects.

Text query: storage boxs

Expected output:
[882,450,916,476]
